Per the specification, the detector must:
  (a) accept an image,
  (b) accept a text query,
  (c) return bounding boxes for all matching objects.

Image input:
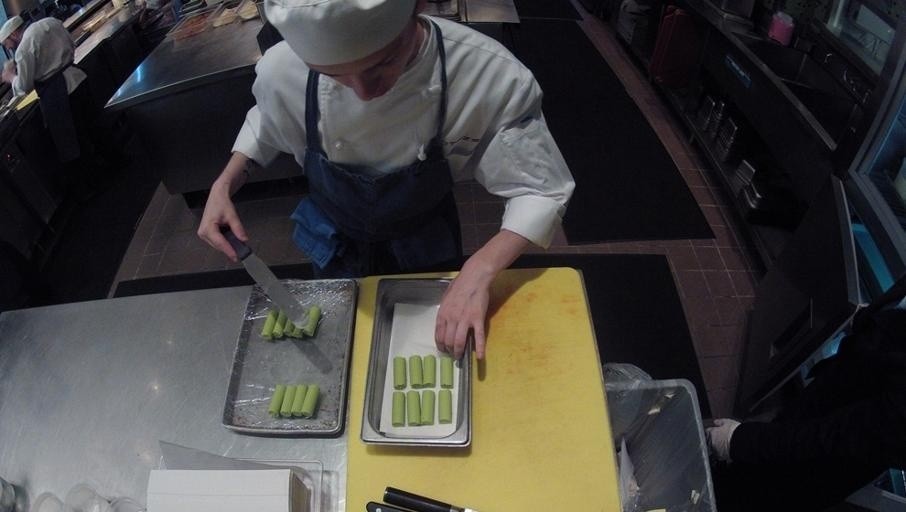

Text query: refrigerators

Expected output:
[729,2,906,418]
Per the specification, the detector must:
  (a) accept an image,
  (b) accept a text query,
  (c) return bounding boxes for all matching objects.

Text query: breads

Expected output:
[392,355,454,426]
[268,384,319,417]
[262,306,320,339]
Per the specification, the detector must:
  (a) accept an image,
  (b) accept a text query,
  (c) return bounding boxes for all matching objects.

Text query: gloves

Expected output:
[705,418,741,463]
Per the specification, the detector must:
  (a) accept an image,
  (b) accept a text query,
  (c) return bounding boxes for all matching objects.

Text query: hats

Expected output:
[0,15,24,44]
[264,0,417,65]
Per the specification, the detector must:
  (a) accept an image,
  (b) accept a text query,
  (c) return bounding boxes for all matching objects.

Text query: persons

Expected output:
[0,15,110,205]
[195,1,576,365]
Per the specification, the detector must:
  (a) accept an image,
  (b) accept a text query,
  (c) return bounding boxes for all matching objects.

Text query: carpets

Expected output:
[502,20,717,246]
[112,251,714,421]
[512,0,584,22]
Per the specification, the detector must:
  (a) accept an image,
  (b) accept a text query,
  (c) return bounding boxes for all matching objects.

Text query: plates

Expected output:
[222,278,358,438]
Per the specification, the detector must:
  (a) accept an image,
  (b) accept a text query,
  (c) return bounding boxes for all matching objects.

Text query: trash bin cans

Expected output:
[605,380,717,512]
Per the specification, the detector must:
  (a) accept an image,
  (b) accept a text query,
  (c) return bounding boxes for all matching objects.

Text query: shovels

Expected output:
[220,225,309,329]
